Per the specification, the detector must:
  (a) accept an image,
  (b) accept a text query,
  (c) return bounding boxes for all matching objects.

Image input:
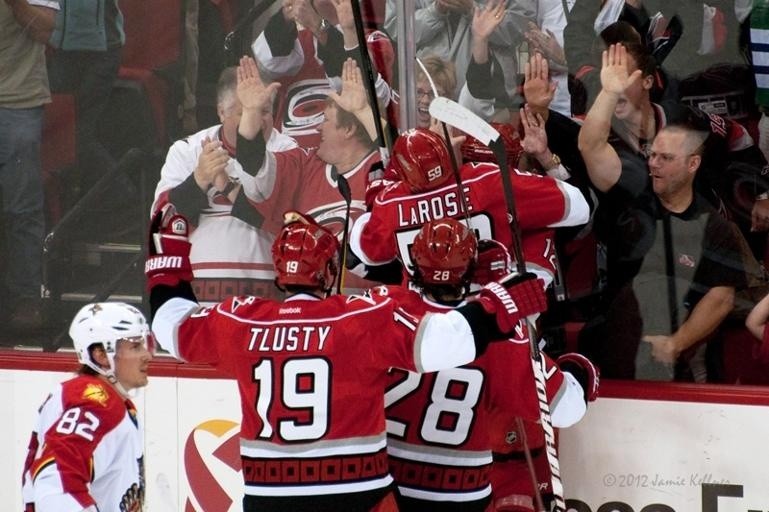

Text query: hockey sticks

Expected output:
[336,173,352,294]
[428,95,566,511]
[415,56,474,231]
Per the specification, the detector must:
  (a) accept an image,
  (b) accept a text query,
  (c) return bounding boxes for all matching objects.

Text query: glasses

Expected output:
[416,88,440,100]
[646,149,697,165]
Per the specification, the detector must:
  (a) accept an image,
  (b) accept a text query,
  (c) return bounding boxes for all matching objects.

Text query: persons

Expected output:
[0,0,769,512]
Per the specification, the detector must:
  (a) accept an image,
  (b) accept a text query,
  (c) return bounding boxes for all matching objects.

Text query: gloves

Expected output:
[144,199,195,292]
[363,160,392,207]
[472,270,551,335]
[556,351,602,402]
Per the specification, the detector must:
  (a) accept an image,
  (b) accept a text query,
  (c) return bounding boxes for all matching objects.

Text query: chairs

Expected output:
[30,0,244,249]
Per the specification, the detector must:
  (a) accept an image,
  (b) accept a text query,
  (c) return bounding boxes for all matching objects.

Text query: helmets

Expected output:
[271,221,339,292]
[460,123,524,169]
[392,128,455,194]
[413,217,510,288]
[68,301,149,378]
